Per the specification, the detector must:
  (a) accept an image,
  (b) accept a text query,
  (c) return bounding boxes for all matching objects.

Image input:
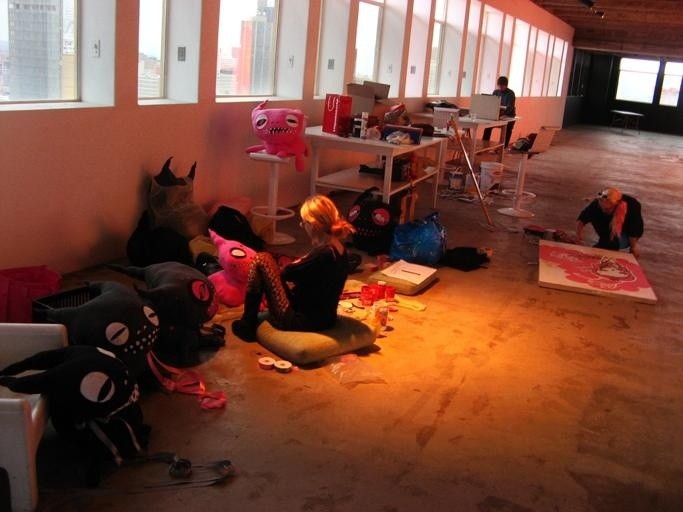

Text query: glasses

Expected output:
[598,191,609,199]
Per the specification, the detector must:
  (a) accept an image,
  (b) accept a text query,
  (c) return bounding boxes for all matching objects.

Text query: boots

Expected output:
[230,288,264,343]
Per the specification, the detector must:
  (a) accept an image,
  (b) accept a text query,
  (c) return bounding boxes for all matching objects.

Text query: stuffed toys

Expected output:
[0,261,226,492]
[245,99,312,171]
[345,185,403,253]
[128,156,269,321]
[0,156,268,492]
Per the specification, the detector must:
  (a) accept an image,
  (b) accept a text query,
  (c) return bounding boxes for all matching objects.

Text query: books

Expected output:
[365,258,441,296]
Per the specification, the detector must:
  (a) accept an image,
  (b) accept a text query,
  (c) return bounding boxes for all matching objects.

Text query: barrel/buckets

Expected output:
[480,162,503,191]
[448,166,464,190]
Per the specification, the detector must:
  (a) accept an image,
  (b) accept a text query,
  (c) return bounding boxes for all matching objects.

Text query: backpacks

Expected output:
[345,186,449,266]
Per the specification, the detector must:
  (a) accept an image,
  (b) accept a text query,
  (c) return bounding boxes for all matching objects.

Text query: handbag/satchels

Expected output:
[442,245,493,272]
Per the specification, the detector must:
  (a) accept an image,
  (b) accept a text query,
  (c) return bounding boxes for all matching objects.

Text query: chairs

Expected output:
[2,319,76,512]
[497,125,563,219]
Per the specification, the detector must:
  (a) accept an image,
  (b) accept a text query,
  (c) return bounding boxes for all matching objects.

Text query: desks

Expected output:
[305,112,519,219]
[610,109,645,134]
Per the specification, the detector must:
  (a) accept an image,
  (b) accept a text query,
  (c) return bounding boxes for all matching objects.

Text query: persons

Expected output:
[230,193,357,343]
[574,186,644,258]
[492,76,516,150]
